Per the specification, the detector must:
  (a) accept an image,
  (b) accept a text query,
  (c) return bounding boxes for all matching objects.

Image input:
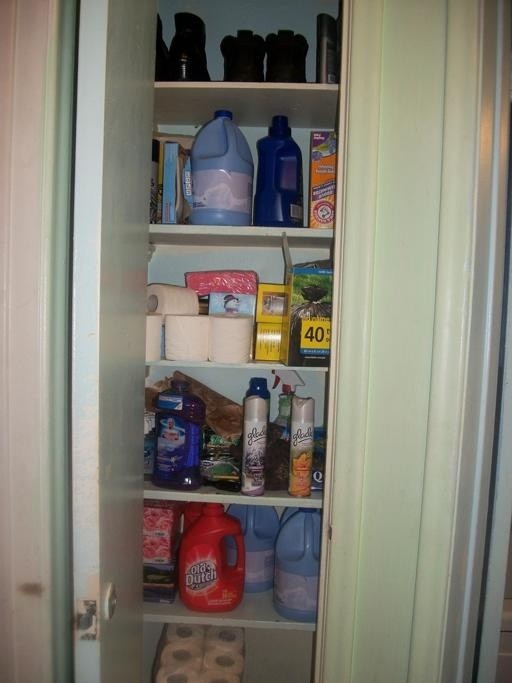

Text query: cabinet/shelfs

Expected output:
[72,0,346,683]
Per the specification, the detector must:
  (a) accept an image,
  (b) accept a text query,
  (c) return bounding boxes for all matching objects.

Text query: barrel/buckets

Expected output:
[189,110,254,226]
[255,117,304,226]
[224,503,280,592]
[179,504,246,612]
[273,509,322,622]
